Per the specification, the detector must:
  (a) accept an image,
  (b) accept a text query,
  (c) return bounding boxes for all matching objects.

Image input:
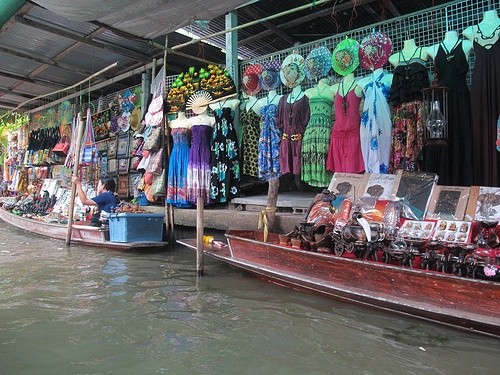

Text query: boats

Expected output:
[0,203,170,250]
[177,228,500,338]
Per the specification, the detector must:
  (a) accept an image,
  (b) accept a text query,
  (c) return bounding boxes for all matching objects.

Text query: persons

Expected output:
[71,176,120,227]
[166,98,240,209]
[239,67,393,188]
[388,9,500,188]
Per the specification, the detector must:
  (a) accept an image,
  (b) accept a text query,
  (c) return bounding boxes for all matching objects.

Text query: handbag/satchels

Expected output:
[26,125,70,166]
[64,143,98,169]
[142,80,166,203]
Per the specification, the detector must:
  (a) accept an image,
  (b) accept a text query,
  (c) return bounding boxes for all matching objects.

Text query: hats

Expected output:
[240,32,393,96]
[107,86,143,137]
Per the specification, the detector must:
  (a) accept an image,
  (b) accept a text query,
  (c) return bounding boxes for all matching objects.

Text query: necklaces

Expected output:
[401,46,420,76]
[288,90,303,117]
[442,39,460,62]
[342,79,357,110]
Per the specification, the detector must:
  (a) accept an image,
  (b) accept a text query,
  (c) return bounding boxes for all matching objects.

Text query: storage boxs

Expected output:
[108,212,166,242]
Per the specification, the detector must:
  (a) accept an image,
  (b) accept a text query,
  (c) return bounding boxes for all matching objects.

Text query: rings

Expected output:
[72,178,74,179]
